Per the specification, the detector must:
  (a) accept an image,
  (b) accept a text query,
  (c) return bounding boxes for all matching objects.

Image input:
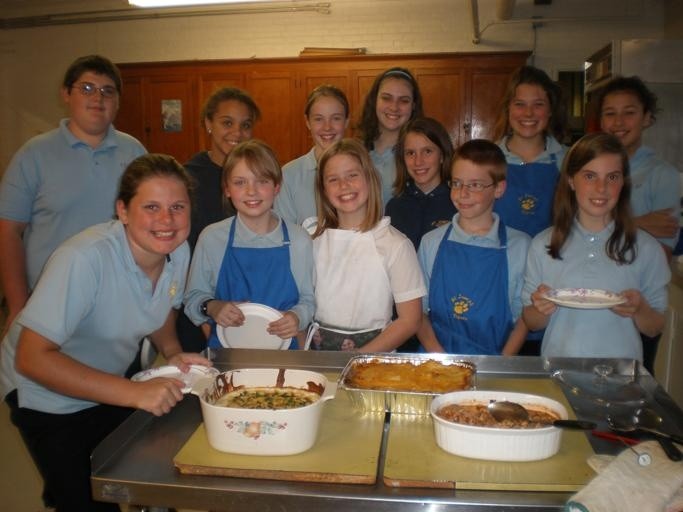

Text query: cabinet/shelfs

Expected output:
[113,50,532,166]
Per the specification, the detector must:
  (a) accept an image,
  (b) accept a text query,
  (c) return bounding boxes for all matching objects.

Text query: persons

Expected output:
[491,66,573,239]
[271,85,350,236]
[0,54,150,339]
[296,138,427,353]
[415,139,533,356]
[348,67,424,207]
[182,140,317,374]
[385,117,459,353]
[595,75,680,374]
[0,152,215,512]
[521,132,673,365]
[175,87,262,354]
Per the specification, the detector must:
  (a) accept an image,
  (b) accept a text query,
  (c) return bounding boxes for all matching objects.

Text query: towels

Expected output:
[565,440,683,512]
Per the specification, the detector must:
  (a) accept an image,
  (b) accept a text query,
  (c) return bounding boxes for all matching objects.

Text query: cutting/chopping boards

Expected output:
[172,371,389,487]
[382,373,600,493]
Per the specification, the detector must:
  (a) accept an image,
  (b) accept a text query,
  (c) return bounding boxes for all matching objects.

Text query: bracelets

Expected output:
[200,299,214,316]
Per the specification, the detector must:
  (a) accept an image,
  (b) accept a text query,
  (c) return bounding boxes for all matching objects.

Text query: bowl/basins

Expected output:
[189,366,339,458]
[428,390,569,462]
[338,353,478,417]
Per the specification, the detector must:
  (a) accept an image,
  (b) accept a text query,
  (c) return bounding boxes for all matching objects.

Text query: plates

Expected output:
[216,301,293,350]
[130,364,219,395]
[541,288,630,309]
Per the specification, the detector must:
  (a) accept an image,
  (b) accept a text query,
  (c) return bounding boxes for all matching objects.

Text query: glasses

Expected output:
[447,179,495,193]
[72,82,117,98]
